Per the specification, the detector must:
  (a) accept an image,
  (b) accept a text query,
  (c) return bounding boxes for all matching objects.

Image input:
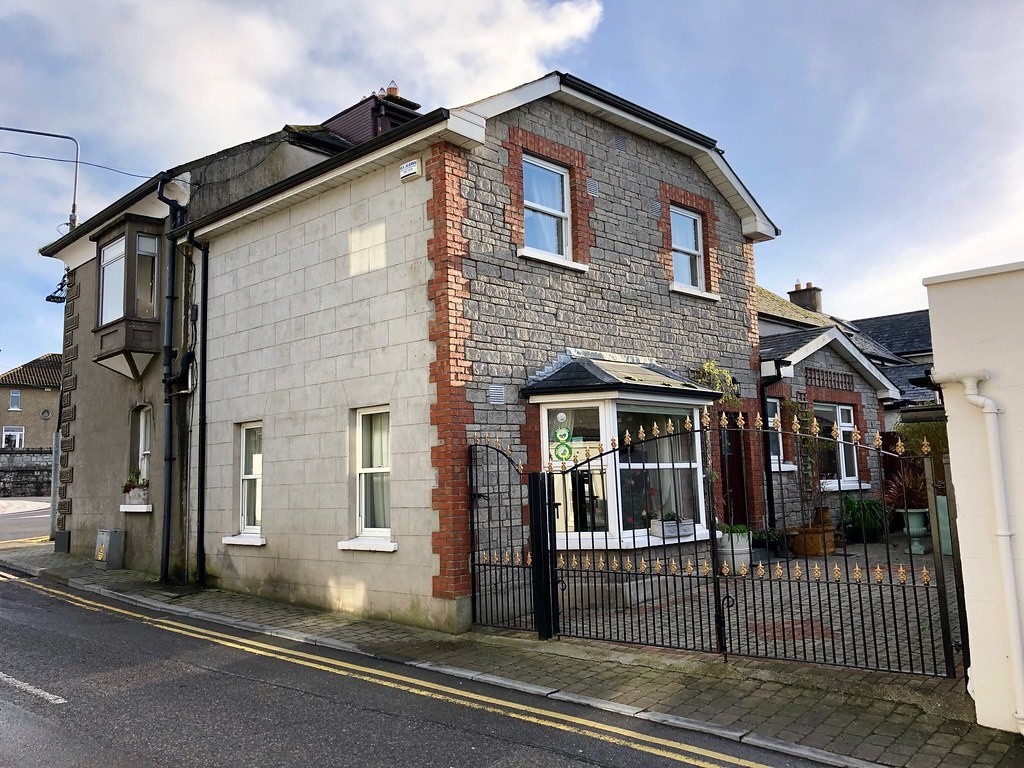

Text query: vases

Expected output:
[815,506,832,526]
[125,488,148,504]
[717,548,751,576]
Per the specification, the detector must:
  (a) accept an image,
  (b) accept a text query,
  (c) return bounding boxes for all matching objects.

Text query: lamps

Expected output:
[732,376,741,398]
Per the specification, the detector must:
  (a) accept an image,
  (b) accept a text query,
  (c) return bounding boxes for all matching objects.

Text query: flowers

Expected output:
[120,466,149,494]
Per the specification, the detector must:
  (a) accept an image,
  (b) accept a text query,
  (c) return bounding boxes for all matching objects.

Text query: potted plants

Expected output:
[753,527,781,553]
[783,400,837,557]
[715,523,753,552]
[843,493,893,544]
[886,465,929,528]
[651,511,693,538]
